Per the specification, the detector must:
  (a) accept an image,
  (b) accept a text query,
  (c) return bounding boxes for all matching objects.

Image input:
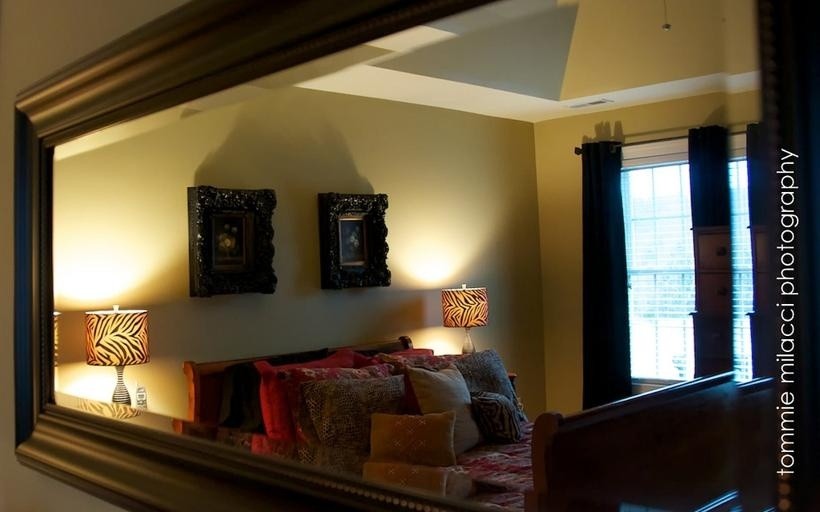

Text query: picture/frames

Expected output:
[186,187,280,299]
[314,193,393,290]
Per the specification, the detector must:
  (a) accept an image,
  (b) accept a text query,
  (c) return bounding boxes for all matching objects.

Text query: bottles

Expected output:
[136,387,147,409]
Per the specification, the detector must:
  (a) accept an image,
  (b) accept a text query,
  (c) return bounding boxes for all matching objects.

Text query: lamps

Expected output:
[82,301,150,405]
[438,281,491,355]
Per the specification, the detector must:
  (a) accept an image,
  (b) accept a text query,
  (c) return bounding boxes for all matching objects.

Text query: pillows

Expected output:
[252,342,528,499]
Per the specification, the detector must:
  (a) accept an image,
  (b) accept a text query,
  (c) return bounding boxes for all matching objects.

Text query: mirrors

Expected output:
[12,1,818,512]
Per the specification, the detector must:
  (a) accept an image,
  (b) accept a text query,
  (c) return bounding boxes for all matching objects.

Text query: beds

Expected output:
[177,344,776,511]
